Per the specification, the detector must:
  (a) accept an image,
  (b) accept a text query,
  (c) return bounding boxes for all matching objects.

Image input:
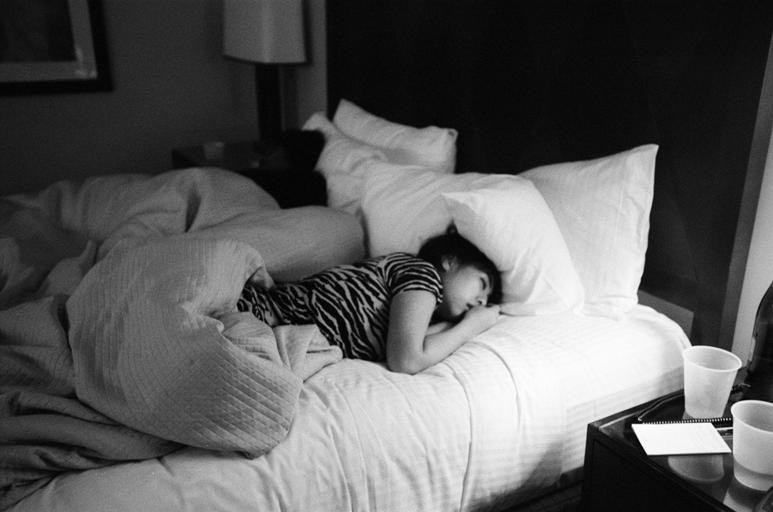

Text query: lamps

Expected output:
[223,0,306,164]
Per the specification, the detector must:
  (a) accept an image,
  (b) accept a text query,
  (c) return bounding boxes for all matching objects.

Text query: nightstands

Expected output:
[577,363,773,511]
[171,128,326,207]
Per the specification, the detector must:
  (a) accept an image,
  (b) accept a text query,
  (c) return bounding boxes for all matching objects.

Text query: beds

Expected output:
[0,0,773,511]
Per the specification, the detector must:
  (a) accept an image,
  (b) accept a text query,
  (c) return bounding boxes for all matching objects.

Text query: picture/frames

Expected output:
[0,0,113,97]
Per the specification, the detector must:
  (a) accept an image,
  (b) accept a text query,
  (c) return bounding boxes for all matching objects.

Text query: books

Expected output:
[629,419,731,458]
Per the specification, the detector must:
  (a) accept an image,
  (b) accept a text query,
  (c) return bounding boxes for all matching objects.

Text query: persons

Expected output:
[236,218,505,375]
